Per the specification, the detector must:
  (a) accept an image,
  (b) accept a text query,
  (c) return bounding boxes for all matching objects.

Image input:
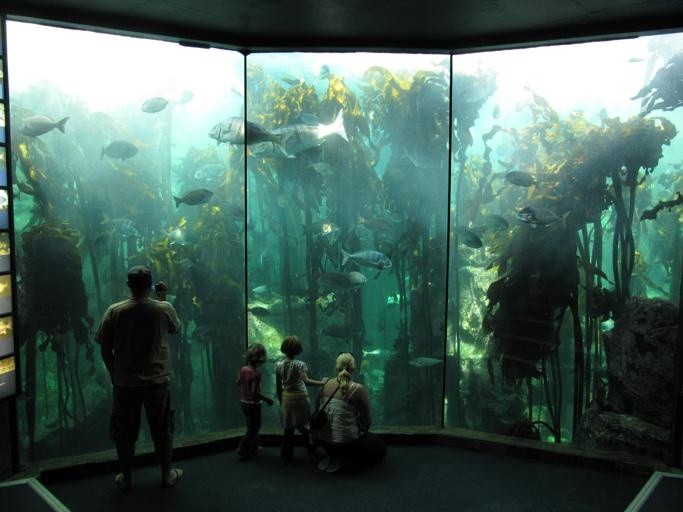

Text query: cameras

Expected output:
[153,281,168,296]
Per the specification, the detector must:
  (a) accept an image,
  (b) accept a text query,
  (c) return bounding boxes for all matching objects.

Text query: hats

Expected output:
[128,264,152,283]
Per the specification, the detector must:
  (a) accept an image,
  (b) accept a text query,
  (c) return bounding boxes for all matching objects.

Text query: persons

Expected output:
[272,336,330,463]
[91,264,188,494]
[233,344,275,458]
[312,351,387,476]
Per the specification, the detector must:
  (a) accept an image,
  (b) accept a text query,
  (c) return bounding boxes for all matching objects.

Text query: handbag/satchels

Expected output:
[310,410,327,430]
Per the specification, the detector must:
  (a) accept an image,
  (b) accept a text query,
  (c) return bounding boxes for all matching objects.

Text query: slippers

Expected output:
[165,468,184,487]
[115,472,132,492]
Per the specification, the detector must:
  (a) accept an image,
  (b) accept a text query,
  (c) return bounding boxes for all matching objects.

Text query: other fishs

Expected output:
[12,93,683,375]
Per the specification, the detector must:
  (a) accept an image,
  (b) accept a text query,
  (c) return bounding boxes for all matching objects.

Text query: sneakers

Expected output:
[318,456,332,471]
[252,446,263,455]
[236,448,248,458]
[326,462,342,473]
